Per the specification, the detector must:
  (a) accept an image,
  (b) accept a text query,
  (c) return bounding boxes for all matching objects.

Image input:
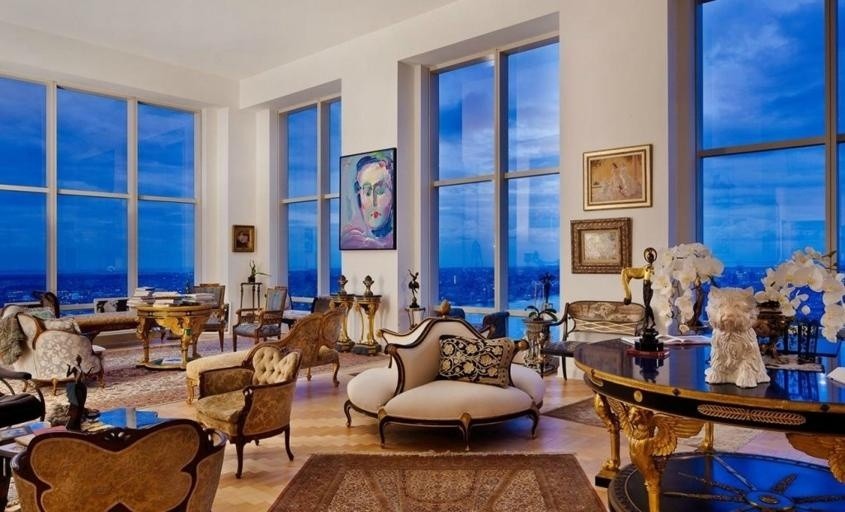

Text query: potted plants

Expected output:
[247,260,271,284]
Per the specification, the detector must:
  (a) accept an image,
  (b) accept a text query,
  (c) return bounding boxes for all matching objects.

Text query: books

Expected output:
[15,418,113,448]
[163,354,181,367]
[621,334,711,346]
[126,284,216,308]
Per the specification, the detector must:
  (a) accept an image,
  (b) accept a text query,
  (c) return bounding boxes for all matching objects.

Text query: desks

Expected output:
[570,331,845,512]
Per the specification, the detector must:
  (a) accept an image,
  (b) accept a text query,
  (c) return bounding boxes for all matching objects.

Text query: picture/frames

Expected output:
[569,217,632,274]
[232,224,255,253]
[338,148,397,251]
[582,143,652,210]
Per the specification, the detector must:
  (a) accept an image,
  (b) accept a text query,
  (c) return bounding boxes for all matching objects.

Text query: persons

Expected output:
[405,268,421,305]
[353,155,393,241]
[541,271,554,303]
[237,230,249,247]
[67,353,94,435]
[605,162,624,194]
